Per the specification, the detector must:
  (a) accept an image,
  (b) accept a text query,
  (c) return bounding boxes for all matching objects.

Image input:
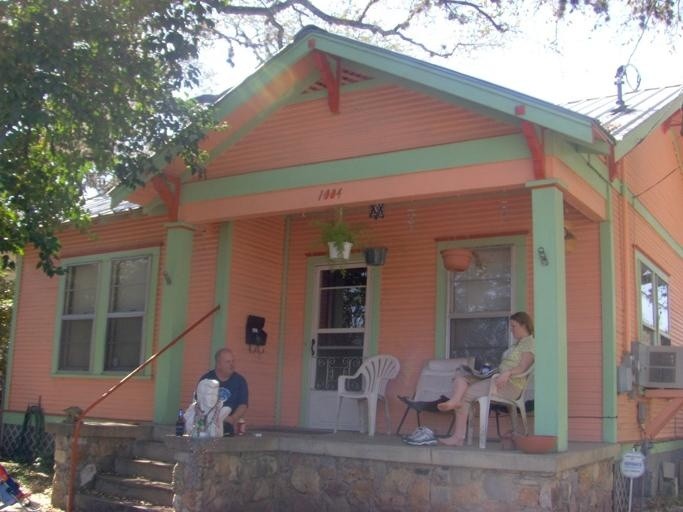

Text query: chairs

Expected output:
[332,354,536,451]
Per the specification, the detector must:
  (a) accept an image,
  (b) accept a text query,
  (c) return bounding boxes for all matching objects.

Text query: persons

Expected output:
[195,347,249,435]
[184,378,232,438]
[436,311,534,446]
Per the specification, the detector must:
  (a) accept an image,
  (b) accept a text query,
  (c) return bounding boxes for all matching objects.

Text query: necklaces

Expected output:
[193,399,224,432]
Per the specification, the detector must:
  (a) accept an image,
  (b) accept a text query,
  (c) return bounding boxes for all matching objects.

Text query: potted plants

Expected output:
[312,208,357,263]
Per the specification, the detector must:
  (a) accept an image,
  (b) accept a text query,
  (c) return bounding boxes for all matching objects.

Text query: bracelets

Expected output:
[509,369,513,376]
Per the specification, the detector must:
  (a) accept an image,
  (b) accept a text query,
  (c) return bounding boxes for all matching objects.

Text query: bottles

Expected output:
[196,410,206,435]
[176,408,184,436]
[237,416,247,435]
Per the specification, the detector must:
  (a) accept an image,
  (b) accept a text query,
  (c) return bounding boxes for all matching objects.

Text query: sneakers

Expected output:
[401,425,439,447]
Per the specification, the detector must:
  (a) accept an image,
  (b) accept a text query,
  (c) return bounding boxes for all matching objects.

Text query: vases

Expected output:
[440,247,475,272]
